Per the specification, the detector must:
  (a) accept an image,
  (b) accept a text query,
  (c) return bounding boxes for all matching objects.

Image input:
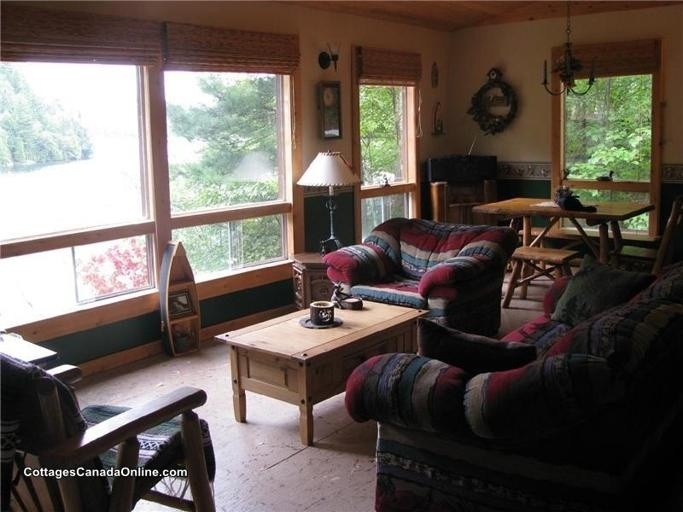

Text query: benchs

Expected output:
[516,225,660,257]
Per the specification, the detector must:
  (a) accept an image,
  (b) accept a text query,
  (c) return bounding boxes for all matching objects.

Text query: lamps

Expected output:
[295,149,365,251]
[542,2,597,99]
[318,38,345,73]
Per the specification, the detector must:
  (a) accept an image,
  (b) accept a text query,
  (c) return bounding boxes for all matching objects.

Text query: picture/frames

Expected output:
[168,289,195,320]
[314,80,345,141]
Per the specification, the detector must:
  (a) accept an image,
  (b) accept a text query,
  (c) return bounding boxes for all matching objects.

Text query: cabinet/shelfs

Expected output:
[425,177,502,225]
[157,241,202,359]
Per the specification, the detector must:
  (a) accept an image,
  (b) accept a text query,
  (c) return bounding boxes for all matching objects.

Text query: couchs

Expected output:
[322,217,521,337]
[346,262,680,512]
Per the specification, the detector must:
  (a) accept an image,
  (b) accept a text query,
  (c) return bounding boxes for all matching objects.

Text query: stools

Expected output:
[502,242,587,312]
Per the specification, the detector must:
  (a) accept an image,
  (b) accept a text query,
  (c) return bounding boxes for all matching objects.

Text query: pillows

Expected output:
[412,317,540,376]
[549,250,622,328]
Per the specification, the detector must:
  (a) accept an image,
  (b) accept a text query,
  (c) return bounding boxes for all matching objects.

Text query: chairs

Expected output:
[0,351,226,512]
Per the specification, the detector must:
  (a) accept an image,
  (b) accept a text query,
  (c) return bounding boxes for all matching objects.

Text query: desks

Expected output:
[289,252,361,310]
[210,295,434,446]
[0,334,58,369]
[471,196,658,259]
